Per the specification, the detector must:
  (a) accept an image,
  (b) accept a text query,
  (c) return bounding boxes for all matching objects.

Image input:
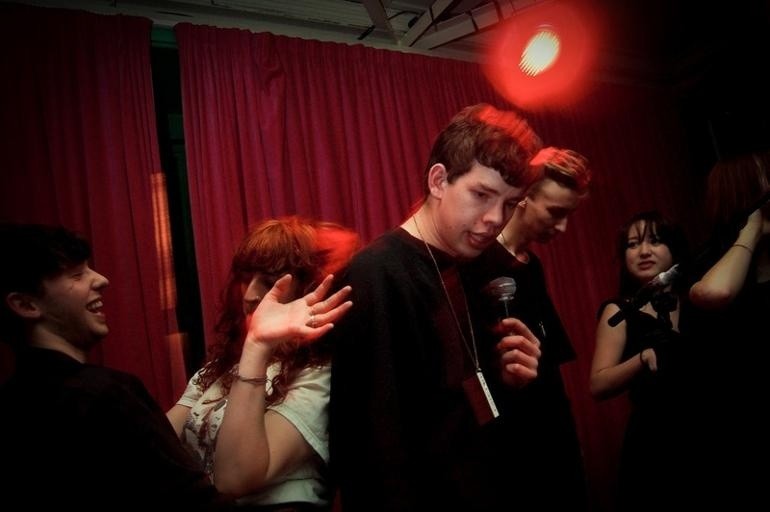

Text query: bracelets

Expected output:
[731,241,753,258]
[228,362,270,387]
[639,349,646,365]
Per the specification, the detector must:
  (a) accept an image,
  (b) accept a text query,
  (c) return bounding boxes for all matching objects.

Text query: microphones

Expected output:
[607,265,675,327]
[485,274,518,338]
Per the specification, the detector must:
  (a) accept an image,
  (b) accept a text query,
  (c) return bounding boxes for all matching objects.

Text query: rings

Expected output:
[311,314,316,327]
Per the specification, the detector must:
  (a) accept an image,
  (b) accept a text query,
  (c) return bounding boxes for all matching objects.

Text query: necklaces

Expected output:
[410,211,502,423]
[498,232,530,264]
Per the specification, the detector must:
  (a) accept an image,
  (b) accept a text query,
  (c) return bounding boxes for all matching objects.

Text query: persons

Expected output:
[0,218,236,509]
[163,212,364,509]
[588,205,739,509]
[324,105,592,510]
[688,145,769,511]
[475,145,591,511]
